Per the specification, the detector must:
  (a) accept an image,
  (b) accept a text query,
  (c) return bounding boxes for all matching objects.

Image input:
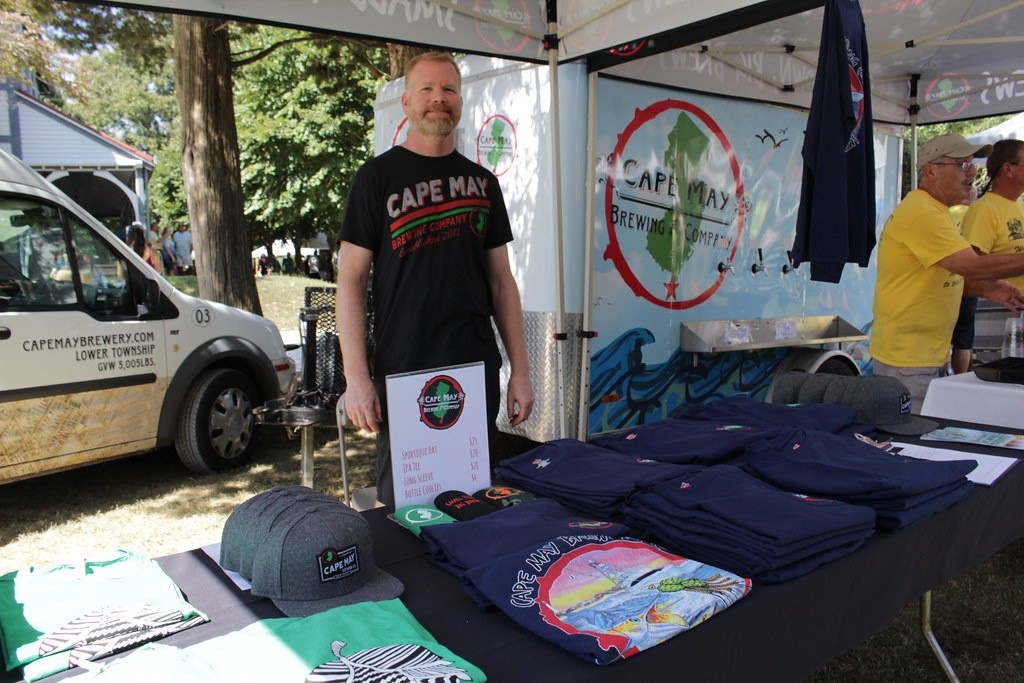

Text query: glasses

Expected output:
[932,161,979,171]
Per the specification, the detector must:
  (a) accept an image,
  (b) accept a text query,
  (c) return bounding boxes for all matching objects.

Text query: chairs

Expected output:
[335,393,385,512]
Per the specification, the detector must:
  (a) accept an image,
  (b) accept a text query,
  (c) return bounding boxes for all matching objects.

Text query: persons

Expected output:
[335,50,534,512]
[869,132,1024,416]
[110,210,344,290]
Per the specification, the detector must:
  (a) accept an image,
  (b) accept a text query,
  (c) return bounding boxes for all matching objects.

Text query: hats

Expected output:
[218,485,403,618]
[916,134,994,173]
[766,370,941,436]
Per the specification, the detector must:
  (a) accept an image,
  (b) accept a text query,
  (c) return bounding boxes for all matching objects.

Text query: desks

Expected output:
[0,414,1024,683]
[919,372,1024,432]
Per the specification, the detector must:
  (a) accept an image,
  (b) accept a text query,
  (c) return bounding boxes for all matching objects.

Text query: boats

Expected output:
[581,555,674,592]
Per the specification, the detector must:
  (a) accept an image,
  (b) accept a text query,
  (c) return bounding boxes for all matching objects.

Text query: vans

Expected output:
[0,147,296,488]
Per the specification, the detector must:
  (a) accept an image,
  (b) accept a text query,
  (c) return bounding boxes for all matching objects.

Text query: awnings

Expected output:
[84,0,1024,441]
